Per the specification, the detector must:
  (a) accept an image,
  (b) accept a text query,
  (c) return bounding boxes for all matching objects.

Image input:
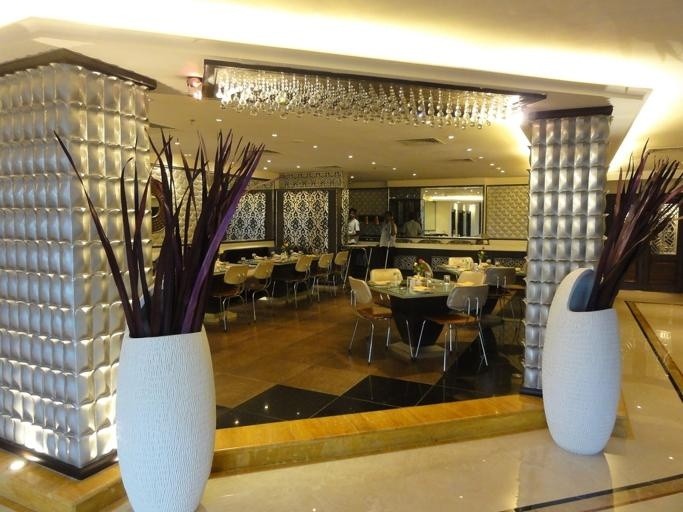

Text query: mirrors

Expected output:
[418,184,487,239]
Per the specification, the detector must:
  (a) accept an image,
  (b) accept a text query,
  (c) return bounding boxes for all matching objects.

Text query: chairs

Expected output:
[212,250,348,334]
[348,255,527,380]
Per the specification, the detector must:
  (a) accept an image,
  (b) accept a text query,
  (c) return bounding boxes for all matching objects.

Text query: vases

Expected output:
[541,267,621,458]
[116,281,217,512]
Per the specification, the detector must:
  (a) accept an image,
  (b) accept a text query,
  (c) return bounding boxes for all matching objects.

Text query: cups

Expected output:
[390,272,450,293]
[240,250,303,263]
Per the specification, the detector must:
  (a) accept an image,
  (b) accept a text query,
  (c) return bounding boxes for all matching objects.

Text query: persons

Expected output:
[379,211,397,245]
[402,213,422,238]
[347,208,360,245]
